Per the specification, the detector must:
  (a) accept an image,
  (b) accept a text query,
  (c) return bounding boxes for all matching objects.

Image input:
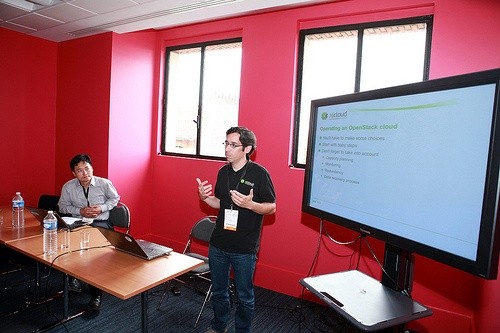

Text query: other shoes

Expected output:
[86,295,102,310]
[70,278,81,294]
[205,327,227,332]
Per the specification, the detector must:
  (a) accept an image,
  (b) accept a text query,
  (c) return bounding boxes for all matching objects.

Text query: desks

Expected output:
[0,208,92,317]
[5,226,204,333]
[298,269,433,333]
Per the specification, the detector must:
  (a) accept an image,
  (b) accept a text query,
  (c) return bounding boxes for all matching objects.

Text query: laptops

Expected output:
[24,205,83,229]
[95,225,173,260]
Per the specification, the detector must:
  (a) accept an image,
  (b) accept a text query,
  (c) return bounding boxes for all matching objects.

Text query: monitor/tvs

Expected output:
[301,66,500,279]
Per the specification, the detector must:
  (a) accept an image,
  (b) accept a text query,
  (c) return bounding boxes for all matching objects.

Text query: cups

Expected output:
[60,228,70,250]
[80,230,89,250]
[0,209,3,226]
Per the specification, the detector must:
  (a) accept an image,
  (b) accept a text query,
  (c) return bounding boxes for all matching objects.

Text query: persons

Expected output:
[196,127,276,333]
[58,154,120,310]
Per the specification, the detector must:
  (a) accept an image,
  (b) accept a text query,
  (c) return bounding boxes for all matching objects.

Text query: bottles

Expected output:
[12,192,24,228]
[42,211,58,255]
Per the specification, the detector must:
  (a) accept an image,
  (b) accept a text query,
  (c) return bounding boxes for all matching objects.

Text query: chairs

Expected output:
[37,195,60,211]
[108,201,131,235]
[155,215,218,329]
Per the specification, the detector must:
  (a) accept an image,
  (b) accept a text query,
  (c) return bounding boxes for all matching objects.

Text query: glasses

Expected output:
[77,167,91,173]
[222,141,244,148]
